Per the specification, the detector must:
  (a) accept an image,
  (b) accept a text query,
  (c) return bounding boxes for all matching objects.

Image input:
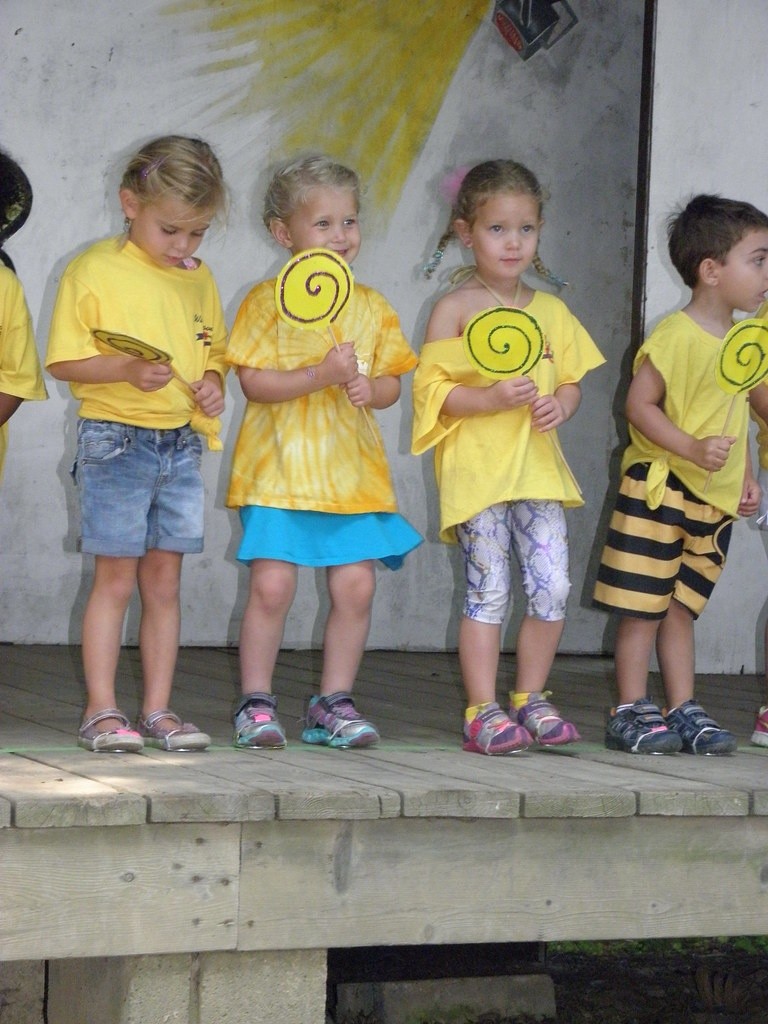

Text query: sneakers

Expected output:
[460,702,533,756]
[302,691,381,748]
[605,696,682,754]
[751,706,768,747]
[137,710,211,752]
[232,692,287,750]
[77,708,144,752]
[509,690,581,746]
[661,699,737,754]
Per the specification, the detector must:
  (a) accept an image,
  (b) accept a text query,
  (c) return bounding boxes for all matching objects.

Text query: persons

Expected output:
[592,195,768,752]
[225,155,420,748]
[45,134,230,751]
[0,149,49,492]
[409,159,606,755]
